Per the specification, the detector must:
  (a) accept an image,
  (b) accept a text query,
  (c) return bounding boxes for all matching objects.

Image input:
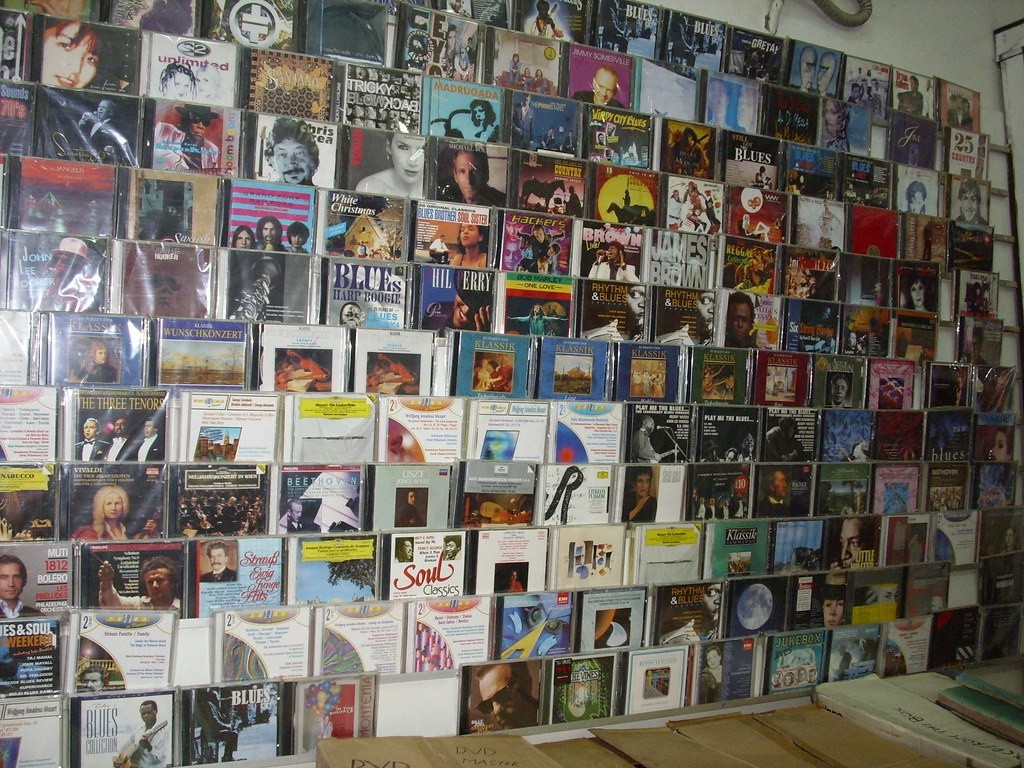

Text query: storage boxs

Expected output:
[316,734,563,768]
[534,726,757,768]
[815,672,1024,768]
[666,704,967,768]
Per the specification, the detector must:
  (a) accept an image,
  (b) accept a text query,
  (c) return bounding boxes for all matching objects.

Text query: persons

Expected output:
[1,2,1022,768]
